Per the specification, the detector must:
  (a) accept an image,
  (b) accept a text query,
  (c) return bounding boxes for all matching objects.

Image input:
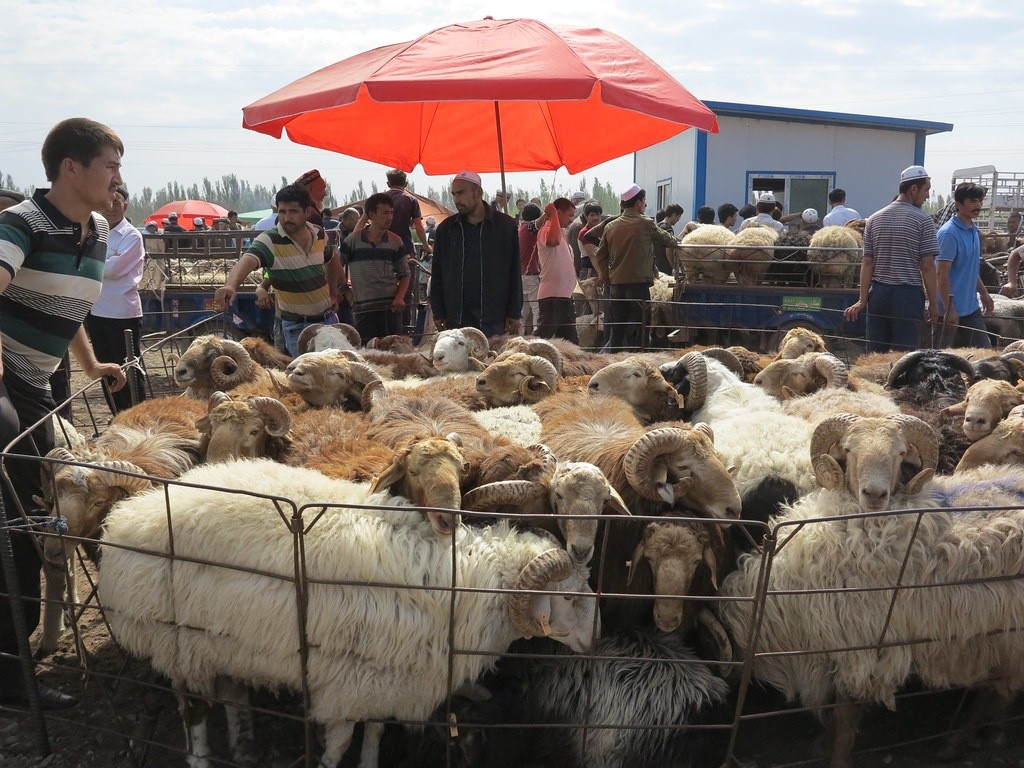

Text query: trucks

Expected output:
[147,244,433,347]
[667,244,867,355]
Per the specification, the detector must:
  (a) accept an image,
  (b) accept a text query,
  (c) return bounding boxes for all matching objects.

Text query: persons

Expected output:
[843,163,940,355]
[935,182,995,348]
[0,180,146,425]
[0,117,129,711]
[136,183,1024,357]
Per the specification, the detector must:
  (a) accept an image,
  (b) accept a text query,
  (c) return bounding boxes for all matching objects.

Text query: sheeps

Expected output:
[978,230,1024,254]
[922,292,1024,350]
[550,219,866,351]
[32,322,1024,768]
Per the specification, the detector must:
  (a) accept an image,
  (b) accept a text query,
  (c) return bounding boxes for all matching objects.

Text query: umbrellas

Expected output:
[143,200,230,231]
[328,188,457,229]
[237,208,273,219]
[241,17,719,217]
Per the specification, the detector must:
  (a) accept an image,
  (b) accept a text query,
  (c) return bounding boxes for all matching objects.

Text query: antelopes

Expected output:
[164,256,263,284]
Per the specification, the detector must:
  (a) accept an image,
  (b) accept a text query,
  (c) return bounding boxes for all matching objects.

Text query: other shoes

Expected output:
[4,682,77,710]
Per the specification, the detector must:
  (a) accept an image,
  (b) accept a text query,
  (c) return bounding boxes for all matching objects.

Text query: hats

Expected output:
[427,216,436,225]
[168,212,177,218]
[454,171,482,186]
[900,165,931,182]
[194,218,203,225]
[295,169,320,186]
[802,208,819,223]
[162,218,170,223]
[758,193,776,204]
[619,184,642,201]
